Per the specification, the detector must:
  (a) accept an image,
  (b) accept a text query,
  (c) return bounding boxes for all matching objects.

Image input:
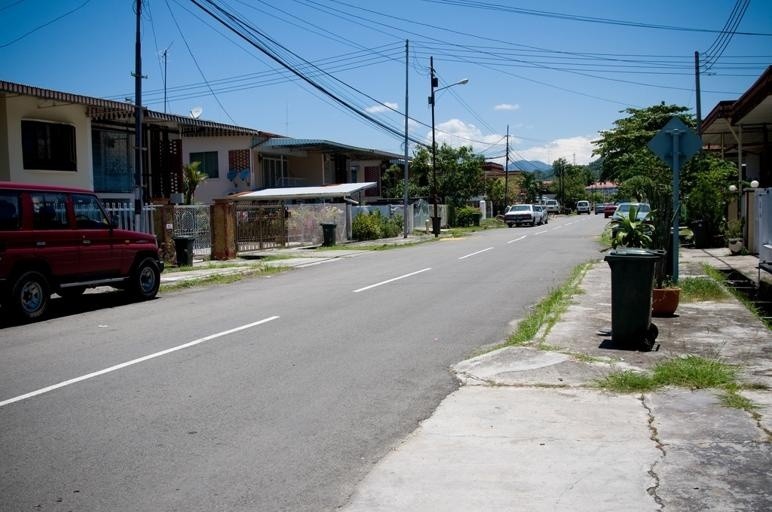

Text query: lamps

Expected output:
[729,185,737,191]
[750,180,759,189]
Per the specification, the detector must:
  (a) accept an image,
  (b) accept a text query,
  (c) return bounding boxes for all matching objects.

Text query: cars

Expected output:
[504,203,541,228]
[604,203,618,218]
[612,203,652,240]
[595,203,604,215]
[534,205,548,226]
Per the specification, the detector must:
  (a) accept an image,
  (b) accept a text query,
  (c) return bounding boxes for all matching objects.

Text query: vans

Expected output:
[576,200,591,215]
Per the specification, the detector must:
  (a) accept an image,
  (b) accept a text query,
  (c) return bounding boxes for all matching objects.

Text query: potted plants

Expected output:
[637,181,682,318]
[726,219,745,254]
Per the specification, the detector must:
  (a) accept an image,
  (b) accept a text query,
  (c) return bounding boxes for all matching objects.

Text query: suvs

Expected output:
[1,181,164,323]
[544,199,560,215]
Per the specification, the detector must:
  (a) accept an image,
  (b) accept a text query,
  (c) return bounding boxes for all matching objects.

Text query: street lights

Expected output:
[428,55,470,237]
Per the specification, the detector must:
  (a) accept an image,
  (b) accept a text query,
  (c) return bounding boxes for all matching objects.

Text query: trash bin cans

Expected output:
[694,220,709,248]
[604,246,667,352]
[430,217,441,234]
[320,223,337,247]
[173,235,195,267]
